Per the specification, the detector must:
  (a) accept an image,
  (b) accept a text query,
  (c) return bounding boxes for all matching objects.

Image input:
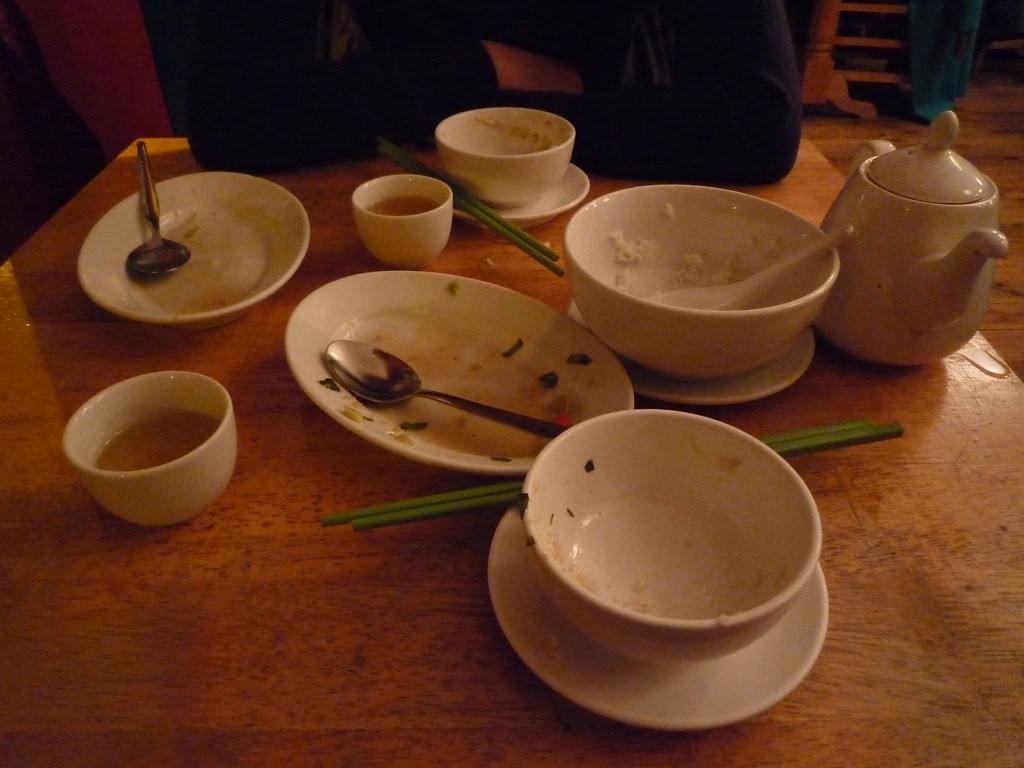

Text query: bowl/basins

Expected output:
[568,186,839,384]
[520,408,821,661]
[433,107,577,209]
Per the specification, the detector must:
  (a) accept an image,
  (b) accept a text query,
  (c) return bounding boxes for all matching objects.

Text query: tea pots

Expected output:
[812,111,1009,369]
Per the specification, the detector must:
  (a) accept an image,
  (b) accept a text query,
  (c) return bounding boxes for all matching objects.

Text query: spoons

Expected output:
[126,142,192,275]
[645,222,854,313]
[323,341,568,440]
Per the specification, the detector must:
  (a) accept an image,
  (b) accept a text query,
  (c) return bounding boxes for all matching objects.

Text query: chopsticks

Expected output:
[377,135,565,278]
[320,418,904,531]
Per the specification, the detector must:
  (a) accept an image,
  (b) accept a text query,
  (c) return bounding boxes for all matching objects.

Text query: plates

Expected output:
[283,271,635,475]
[488,504,827,733]
[77,172,310,331]
[442,163,590,232]
[568,296,817,405]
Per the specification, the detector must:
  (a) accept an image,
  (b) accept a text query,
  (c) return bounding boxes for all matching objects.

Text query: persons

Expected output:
[181,0,801,186]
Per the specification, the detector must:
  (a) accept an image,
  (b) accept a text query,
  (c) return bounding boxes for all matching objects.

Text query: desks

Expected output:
[0,138,1024,768]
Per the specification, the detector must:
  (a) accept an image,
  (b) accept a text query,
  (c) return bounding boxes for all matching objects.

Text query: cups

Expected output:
[62,371,238,528]
[352,175,454,271]
